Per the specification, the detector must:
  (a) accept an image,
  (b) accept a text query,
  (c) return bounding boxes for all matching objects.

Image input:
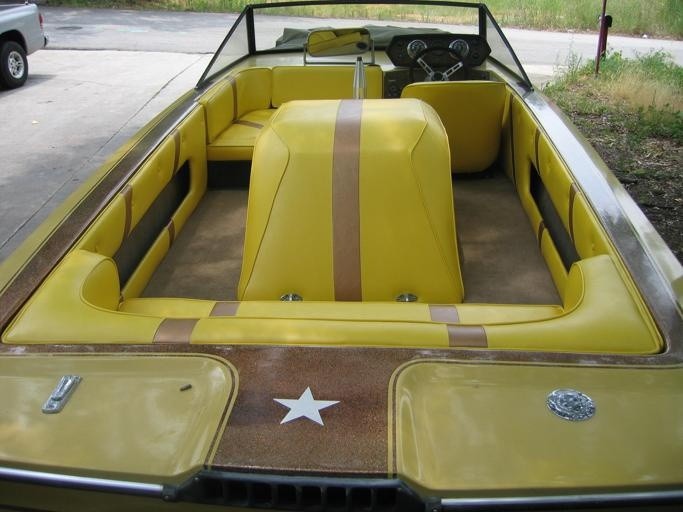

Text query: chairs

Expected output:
[204,66,384,190]
[400,80,508,174]
[239,98,466,303]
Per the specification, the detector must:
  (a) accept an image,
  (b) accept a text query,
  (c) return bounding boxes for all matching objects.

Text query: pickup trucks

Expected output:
[0,0,49,89]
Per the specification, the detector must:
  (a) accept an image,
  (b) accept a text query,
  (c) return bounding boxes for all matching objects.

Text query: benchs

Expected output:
[0,248,664,356]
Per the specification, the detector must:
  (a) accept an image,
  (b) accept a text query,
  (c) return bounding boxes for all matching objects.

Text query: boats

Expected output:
[0,0,683,511]
